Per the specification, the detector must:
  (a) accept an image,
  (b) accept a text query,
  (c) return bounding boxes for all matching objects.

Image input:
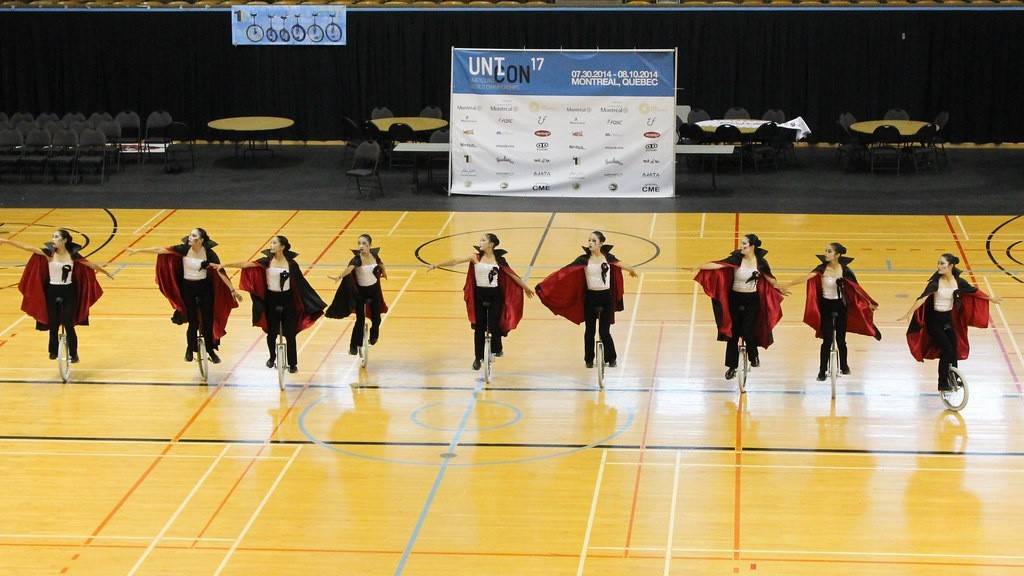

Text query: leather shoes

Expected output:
[725,369,736,379]
[748,355,759,366]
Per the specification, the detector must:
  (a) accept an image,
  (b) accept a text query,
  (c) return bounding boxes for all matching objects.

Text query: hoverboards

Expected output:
[479,302,500,385]
[51,297,80,383]
[591,307,615,385]
[734,305,756,395]
[824,310,845,400]
[938,324,968,413]
[357,296,371,369]
[270,307,291,391]
[189,296,220,381]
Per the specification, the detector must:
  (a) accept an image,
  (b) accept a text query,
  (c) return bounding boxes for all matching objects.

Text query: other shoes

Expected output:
[209,351,220,363]
[290,365,297,373]
[938,385,953,390]
[350,344,357,355]
[50,353,58,359]
[608,360,616,367]
[71,355,79,363]
[473,360,480,370]
[586,361,593,368]
[267,359,274,368]
[370,328,376,344]
[818,370,826,380]
[841,365,850,374]
[186,347,193,361]
[495,351,503,356]
[957,381,962,386]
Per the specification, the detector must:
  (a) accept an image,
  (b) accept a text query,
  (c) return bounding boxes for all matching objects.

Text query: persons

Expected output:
[125,228,244,363]
[421,233,534,370]
[682,234,794,381]
[209,235,328,373]
[897,253,1003,391]
[0,229,114,363]
[535,230,639,368]
[324,233,388,355]
[774,243,882,382]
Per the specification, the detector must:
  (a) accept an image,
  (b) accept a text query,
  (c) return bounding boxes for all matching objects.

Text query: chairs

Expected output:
[0,104,951,198]
